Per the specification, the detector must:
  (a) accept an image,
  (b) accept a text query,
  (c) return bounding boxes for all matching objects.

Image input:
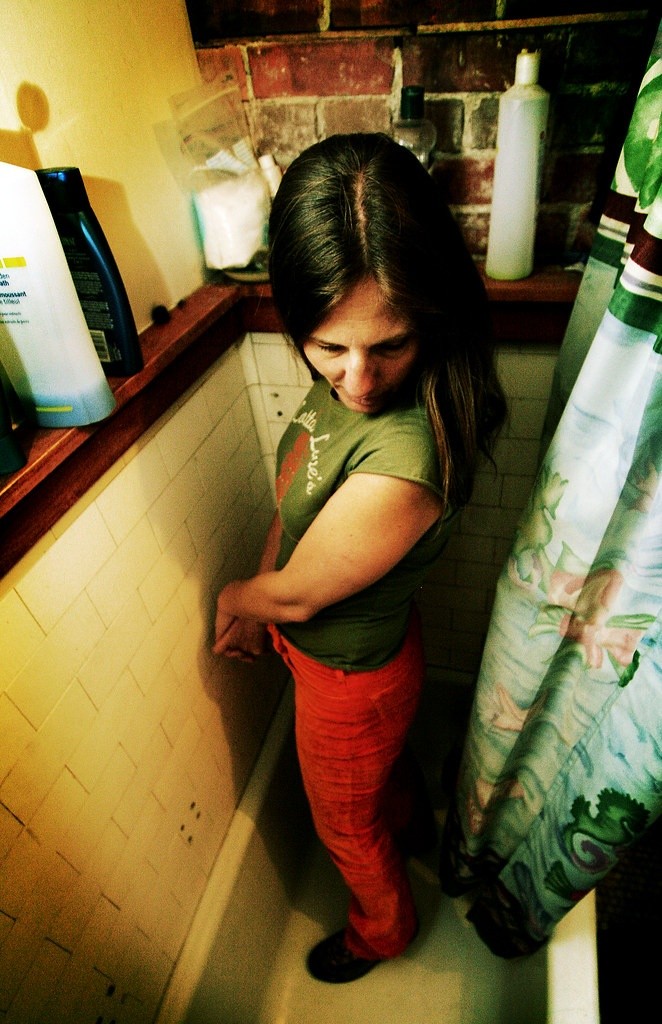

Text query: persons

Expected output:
[212,132,509,983]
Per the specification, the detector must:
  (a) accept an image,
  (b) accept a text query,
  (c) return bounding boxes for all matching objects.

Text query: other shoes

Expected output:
[305,913,422,985]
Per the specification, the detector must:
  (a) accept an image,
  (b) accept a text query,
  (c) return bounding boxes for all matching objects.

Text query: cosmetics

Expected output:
[259,154,282,199]
[394,85,440,177]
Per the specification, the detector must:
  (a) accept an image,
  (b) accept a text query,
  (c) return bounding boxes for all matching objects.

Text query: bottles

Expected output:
[391,85,437,172]
[484,48,550,278]
